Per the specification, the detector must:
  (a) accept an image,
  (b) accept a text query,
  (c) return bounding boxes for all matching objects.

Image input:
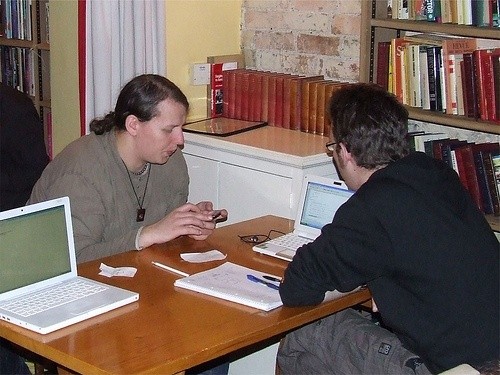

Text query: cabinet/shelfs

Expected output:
[181,144,344,231]
[359,0,500,232]
[0,0,41,116]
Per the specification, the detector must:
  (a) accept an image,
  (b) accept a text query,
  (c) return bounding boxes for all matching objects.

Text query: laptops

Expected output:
[252,173,355,261]
[0,196,140,335]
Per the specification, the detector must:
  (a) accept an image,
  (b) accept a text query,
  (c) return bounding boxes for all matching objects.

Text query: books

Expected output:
[0,0,50,158]
[207,0,500,217]
[175,262,285,312]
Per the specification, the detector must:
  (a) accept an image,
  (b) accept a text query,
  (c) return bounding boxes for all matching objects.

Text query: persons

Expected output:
[23,75,227,266]
[0,83,50,213]
[278,85,500,375]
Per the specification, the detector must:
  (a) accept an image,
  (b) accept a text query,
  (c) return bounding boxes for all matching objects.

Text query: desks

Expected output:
[0,214,374,374]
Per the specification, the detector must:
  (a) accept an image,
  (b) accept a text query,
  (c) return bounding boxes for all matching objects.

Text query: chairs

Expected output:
[0,81,51,212]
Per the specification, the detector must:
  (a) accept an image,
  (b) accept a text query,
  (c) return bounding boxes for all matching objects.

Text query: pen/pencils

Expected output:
[263,276,281,282]
[152,261,189,277]
[247,275,279,290]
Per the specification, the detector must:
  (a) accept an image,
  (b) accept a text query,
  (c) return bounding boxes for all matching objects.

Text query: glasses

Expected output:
[326,141,350,153]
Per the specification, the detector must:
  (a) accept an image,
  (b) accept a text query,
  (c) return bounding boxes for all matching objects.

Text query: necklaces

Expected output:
[120,157,151,223]
